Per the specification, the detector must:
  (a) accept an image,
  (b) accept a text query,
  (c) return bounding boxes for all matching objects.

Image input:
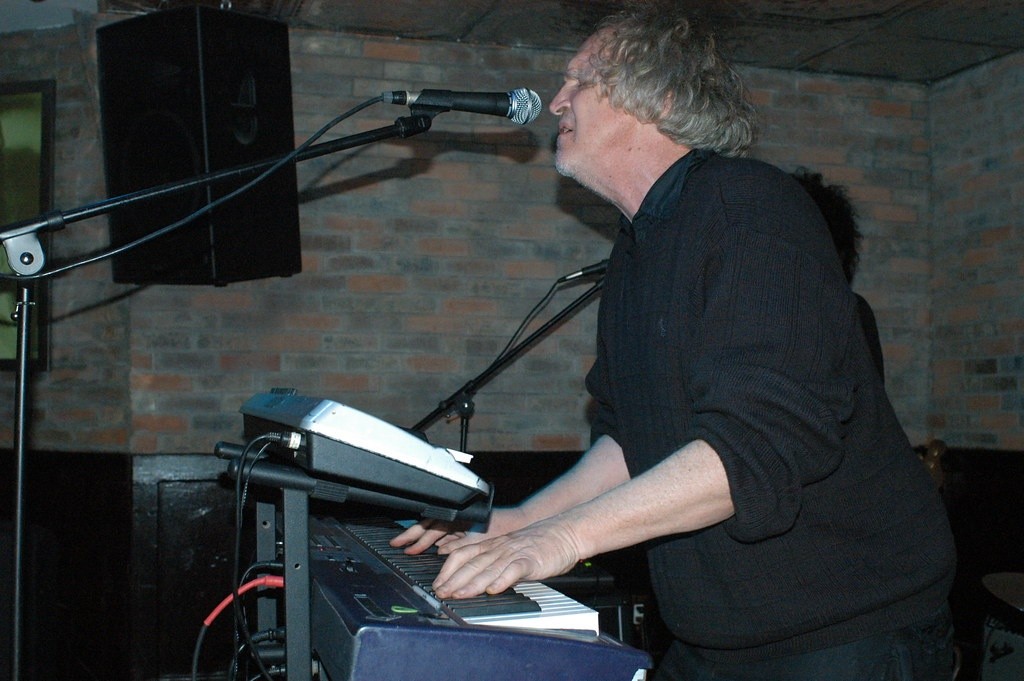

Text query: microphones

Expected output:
[383,85,542,125]
[558,259,610,284]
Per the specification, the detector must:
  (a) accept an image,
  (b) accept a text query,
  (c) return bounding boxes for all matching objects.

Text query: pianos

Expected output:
[290,513,655,680]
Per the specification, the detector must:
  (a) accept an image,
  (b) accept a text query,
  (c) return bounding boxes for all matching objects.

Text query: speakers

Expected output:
[96,3,303,286]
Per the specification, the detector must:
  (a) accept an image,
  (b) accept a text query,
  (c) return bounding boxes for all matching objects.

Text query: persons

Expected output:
[385,5,960,681]
